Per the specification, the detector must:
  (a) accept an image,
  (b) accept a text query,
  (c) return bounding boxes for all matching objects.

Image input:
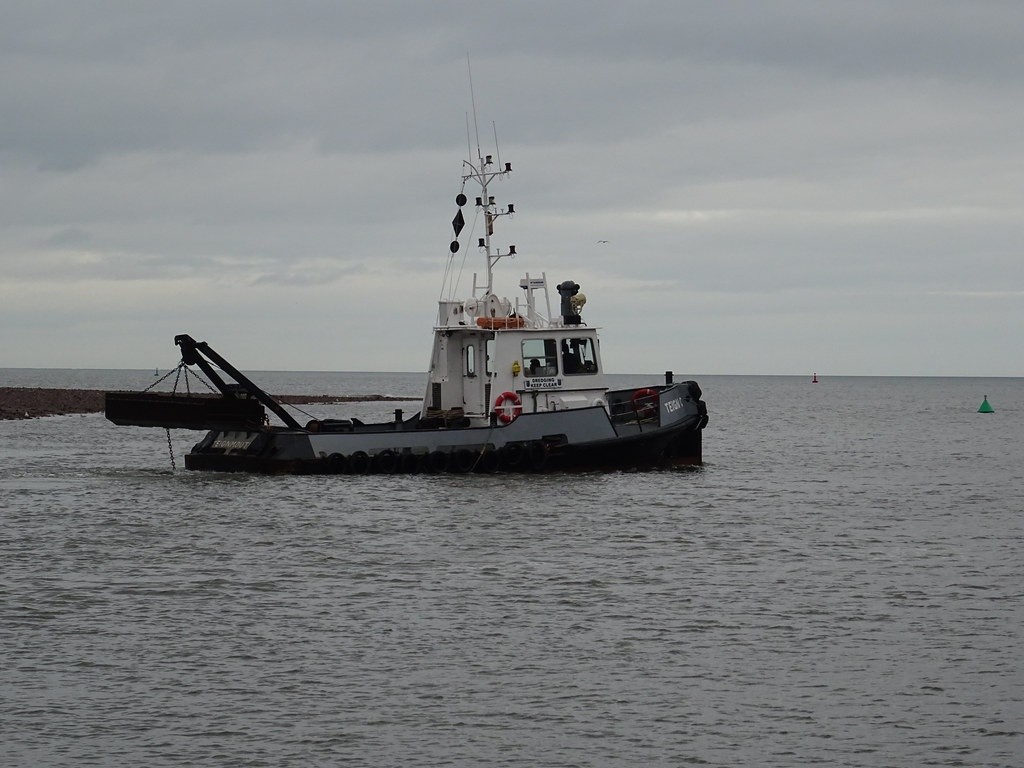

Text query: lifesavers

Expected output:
[323,442,525,474]
[635,389,660,396]
[495,391,521,425]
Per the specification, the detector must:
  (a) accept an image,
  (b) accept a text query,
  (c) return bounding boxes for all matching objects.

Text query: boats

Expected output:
[170,49,711,475]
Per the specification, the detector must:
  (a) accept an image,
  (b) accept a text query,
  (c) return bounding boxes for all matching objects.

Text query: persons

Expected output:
[530,359,540,375]
[562,344,580,374]
